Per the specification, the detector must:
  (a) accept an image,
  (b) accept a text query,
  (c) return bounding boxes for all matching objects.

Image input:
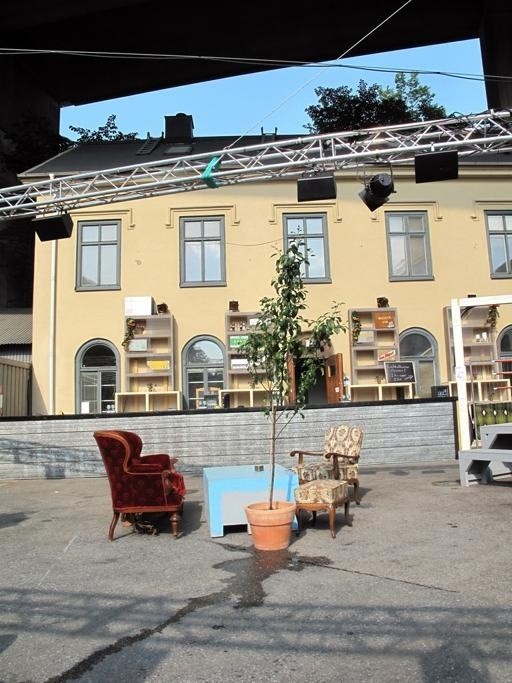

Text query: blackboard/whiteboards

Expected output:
[384,361,417,384]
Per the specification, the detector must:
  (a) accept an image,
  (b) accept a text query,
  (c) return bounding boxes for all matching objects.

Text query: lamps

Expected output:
[298,148,460,212]
[32,208,73,241]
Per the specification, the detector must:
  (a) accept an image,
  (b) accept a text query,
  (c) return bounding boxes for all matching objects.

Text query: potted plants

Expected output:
[238,236,347,551]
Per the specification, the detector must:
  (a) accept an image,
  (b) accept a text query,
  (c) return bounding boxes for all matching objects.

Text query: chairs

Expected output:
[288,424,363,506]
[93,430,186,541]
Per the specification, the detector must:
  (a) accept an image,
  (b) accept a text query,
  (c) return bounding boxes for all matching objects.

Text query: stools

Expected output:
[293,478,353,538]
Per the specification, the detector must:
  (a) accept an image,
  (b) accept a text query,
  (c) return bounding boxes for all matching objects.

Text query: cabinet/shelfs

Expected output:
[443,305,511,404]
[225,311,284,408]
[348,306,413,402]
[114,314,183,413]
